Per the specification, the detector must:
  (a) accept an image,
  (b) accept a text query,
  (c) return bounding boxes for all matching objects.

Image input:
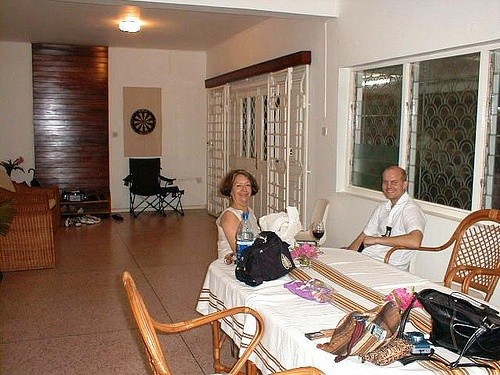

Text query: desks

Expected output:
[194,245,500,375]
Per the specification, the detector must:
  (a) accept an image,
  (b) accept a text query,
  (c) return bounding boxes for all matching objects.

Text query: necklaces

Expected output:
[239,204,247,207]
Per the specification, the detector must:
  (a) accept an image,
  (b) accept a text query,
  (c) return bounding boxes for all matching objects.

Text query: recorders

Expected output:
[62,190,88,202]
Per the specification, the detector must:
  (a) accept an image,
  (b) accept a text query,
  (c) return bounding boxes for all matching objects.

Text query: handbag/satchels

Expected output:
[361,336,413,366]
[316,299,402,362]
[236,231,296,286]
[397,288,500,370]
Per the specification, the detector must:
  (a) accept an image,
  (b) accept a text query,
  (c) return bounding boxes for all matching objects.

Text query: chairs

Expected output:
[0,180,62,271]
[294,199,330,247]
[122,158,187,218]
[122,271,327,375]
[384,209,500,303]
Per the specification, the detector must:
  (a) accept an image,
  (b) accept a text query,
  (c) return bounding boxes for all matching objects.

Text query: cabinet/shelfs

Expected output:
[59,199,111,219]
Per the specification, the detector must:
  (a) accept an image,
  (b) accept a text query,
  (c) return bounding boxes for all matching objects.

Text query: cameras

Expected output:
[404,332,431,354]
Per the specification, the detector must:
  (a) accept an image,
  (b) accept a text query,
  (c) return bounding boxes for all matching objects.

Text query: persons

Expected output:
[216,170,262,259]
[347,165,426,272]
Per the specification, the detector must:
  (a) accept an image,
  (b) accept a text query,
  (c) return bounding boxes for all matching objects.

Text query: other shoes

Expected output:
[112,213,124,221]
[63,212,101,226]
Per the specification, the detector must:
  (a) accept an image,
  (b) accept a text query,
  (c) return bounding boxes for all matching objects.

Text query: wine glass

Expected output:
[312,221,325,255]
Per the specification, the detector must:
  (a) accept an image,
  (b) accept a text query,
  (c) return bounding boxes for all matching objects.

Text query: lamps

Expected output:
[118,20,141,33]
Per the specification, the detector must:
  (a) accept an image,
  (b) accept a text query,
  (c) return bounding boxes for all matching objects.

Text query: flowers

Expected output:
[290,243,323,268]
[385,286,424,315]
[0,155,26,176]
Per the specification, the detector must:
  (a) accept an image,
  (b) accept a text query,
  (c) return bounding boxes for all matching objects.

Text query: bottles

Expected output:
[236,212,254,265]
